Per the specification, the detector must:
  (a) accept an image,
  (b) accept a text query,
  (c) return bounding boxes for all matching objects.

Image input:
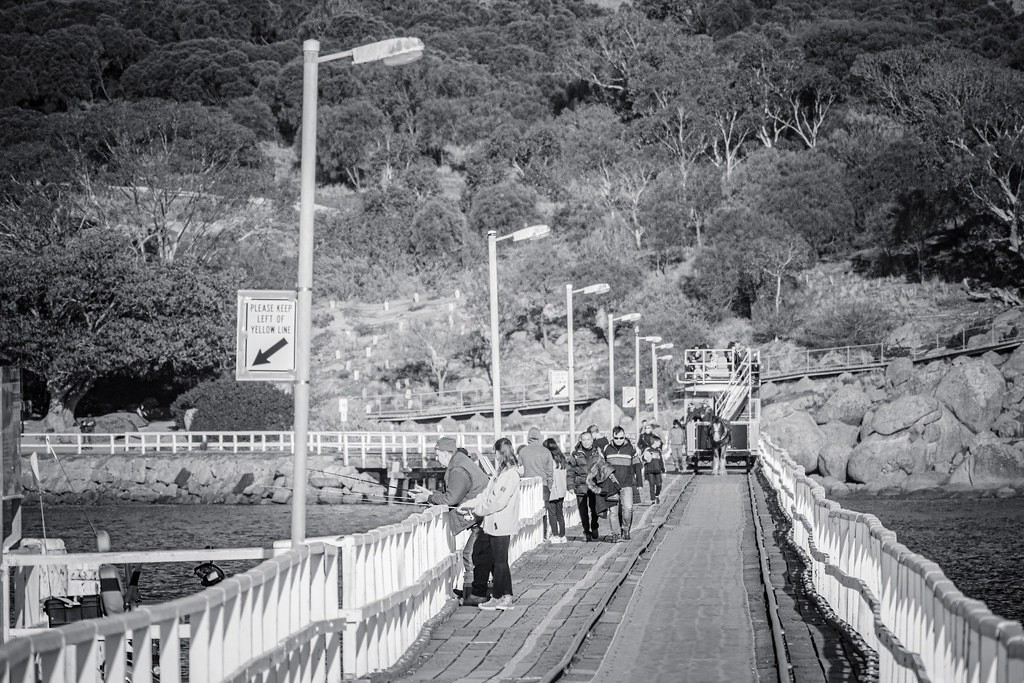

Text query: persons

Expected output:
[517,427,567,543]
[637,420,666,505]
[83,414,95,444]
[136,405,148,418]
[566,424,643,542]
[667,403,730,476]
[685,343,712,380]
[725,340,747,381]
[406,438,491,606]
[456,438,524,609]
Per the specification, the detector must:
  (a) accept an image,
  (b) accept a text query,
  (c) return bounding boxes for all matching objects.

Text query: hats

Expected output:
[435,437,456,451]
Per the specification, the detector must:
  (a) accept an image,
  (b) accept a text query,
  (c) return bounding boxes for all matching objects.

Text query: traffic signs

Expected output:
[236,288,298,382]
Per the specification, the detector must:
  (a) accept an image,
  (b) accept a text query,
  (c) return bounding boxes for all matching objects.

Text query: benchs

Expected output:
[705,354,729,378]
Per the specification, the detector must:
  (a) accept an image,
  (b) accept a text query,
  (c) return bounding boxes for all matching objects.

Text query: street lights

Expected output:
[487,224,551,473]
[651,342,673,426]
[634,336,662,443]
[607,312,642,440]
[289,35,424,546]
[566,282,610,454]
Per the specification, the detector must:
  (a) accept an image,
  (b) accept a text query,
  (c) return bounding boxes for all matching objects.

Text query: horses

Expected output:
[706,414,733,476]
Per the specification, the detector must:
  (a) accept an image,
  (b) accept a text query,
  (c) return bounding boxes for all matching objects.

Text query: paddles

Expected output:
[29,451,48,555]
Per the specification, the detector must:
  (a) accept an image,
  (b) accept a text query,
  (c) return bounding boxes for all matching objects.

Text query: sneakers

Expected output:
[464,594,488,605]
[479,595,515,609]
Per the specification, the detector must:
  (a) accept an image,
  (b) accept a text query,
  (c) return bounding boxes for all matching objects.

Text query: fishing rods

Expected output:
[304,495,457,510]
[44,438,97,537]
[306,466,414,494]
[260,484,412,501]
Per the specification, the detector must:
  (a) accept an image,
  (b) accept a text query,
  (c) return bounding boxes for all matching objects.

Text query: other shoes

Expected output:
[453,589,463,597]
[612,534,622,543]
[586,532,592,542]
[624,530,630,539]
[592,529,598,539]
[548,534,567,544]
[680,465,683,470]
[675,468,678,472]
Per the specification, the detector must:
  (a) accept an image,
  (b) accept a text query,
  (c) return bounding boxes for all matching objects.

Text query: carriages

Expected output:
[676,346,762,474]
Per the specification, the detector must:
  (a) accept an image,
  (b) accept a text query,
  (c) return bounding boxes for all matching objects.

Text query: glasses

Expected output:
[613,435,624,440]
